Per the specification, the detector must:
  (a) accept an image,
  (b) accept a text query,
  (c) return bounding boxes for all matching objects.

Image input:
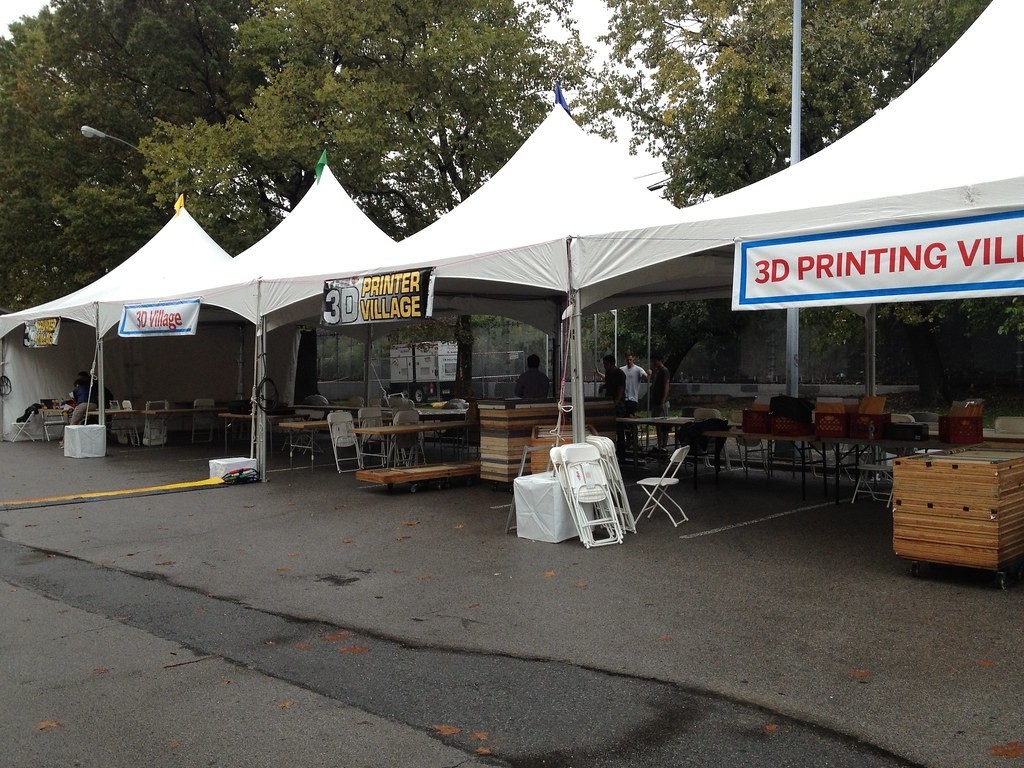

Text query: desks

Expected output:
[217,411,480,493]
[38,405,229,447]
[616,415,984,507]
[969,441,1024,452]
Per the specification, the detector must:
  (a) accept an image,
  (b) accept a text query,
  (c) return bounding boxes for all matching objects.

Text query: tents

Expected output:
[0,4,1022,483]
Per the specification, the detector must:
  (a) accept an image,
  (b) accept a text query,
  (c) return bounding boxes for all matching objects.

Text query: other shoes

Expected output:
[652,445,667,453]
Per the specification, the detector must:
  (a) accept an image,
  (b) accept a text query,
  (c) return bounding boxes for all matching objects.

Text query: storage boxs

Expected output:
[938,415,984,444]
[769,413,812,435]
[742,410,770,434]
[814,413,848,439]
[848,412,891,441]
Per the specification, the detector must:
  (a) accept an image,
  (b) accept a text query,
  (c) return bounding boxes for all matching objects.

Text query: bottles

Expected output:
[52,398,64,409]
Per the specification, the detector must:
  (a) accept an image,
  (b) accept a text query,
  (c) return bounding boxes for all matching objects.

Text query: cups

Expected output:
[663,407,668,418]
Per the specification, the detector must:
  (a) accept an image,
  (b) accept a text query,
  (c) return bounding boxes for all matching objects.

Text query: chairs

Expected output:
[849,445,906,508]
[109,399,141,446]
[504,432,637,548]
[280,395,478,474]
[142,399,170,448]
[41,408,69,442]
[634,445,691,528]
[10,409,36,443]
[693,407,733,469]
[190,398,215,444]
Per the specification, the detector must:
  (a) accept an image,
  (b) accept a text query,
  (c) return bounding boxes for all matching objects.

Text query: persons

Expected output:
[60,379,97,449]
[77,370,114,447]
[514,353,550,403]
[593,351,653,450]
[597,354,627,455]
[649,354,671,451]
